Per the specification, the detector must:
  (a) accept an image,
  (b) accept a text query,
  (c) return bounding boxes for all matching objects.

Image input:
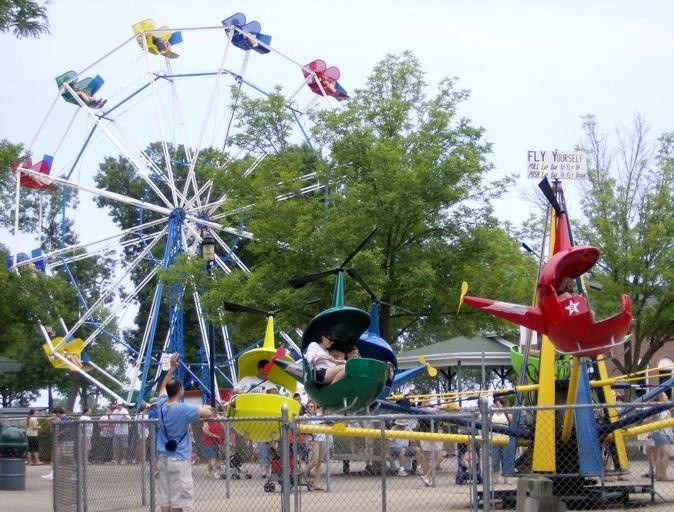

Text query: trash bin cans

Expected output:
[0,427,28,491]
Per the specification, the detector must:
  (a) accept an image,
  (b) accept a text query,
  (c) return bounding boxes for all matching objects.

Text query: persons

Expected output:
[227,18,258,48]
[62,76,93,102]
[651,391,673,482]
[309,62,333,91]
[347,344,360,360]
[135,22,166,53]
[87,95,107,104]
[78,407,94,465]
[96,396,158,465]
[48,350,84,368]
[157,352,213,512]
[386,389,516,486]
[557,276,576,302]
[25,409,44,466]
[200,405,226,480]
[17,167,48,186]
[230,357,327,493]
[41,406,77,482]
[304,330,346,385]
[640,447,654,478]
[329,348,345,362]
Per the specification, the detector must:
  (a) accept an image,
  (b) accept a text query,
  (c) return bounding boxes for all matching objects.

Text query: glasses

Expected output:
[325,337,335,342]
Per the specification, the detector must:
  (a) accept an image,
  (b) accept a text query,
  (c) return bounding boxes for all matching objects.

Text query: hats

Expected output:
[321,331,340,340]
[395,418,411,426]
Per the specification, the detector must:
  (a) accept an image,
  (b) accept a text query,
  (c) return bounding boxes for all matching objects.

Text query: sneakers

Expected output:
[397,470,408,477]
[421,476,430,487]
[315,485,325,490]
[302,467,308,478]
[415,470,424,475]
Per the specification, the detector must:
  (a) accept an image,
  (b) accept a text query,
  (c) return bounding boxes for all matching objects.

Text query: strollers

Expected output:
[218,439,251,480]
[454,439,482,485]
[262,423,316,491]
